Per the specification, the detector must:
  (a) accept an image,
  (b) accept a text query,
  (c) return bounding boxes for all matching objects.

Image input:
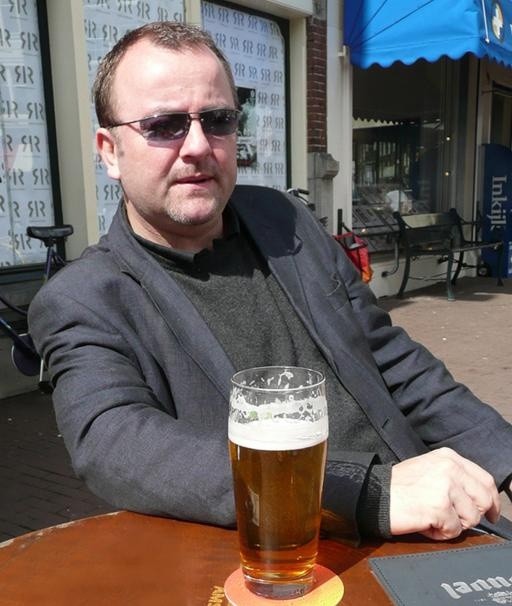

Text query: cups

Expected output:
[226,366,328,601]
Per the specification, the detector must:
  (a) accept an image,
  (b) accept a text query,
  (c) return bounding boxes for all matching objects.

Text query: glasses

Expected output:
[101,109,243,141]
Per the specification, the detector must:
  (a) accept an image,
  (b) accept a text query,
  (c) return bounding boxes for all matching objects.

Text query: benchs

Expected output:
[392,207,507,301]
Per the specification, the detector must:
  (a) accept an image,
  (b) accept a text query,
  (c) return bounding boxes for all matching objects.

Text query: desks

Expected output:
[0,511,503,606]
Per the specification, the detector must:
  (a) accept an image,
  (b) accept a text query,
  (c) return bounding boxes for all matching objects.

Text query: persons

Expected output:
[378,180,410,218]
[28,25,510,540]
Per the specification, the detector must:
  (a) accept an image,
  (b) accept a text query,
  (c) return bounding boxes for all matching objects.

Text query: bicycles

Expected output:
[1,223,75,396]
